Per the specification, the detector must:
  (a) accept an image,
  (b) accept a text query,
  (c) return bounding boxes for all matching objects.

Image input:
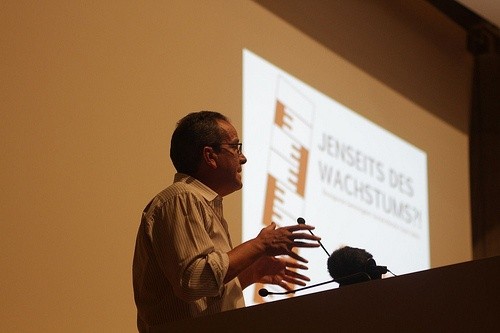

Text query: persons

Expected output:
[133,110,321,333]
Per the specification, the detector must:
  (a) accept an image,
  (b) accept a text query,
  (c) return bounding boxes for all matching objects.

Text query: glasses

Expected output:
[207,142,242,153]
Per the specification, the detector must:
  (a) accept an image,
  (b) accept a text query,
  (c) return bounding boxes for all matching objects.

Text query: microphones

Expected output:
[327,246,381,286]
[297,217,330,257]
[258,272,372,297]
